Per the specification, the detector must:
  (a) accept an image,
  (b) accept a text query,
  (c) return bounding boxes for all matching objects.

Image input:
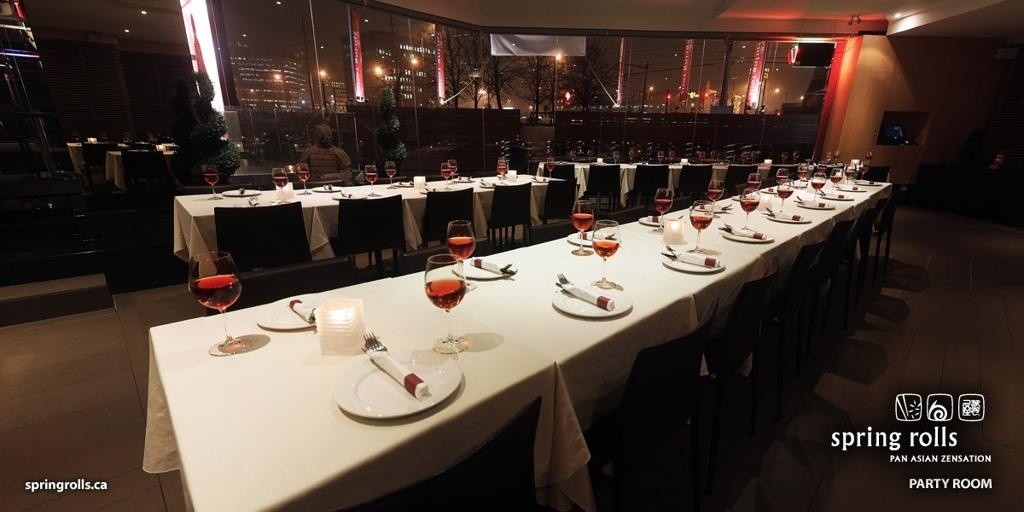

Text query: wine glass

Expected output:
[188,150,872,354]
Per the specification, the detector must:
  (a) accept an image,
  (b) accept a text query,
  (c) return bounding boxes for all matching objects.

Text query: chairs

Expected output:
[64,135,895,512]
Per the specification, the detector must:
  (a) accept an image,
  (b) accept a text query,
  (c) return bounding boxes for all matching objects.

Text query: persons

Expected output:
[301,124,353,181]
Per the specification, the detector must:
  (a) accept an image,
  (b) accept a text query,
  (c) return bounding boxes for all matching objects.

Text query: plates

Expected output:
[858,180,882,186]
[335,348,465,419]
